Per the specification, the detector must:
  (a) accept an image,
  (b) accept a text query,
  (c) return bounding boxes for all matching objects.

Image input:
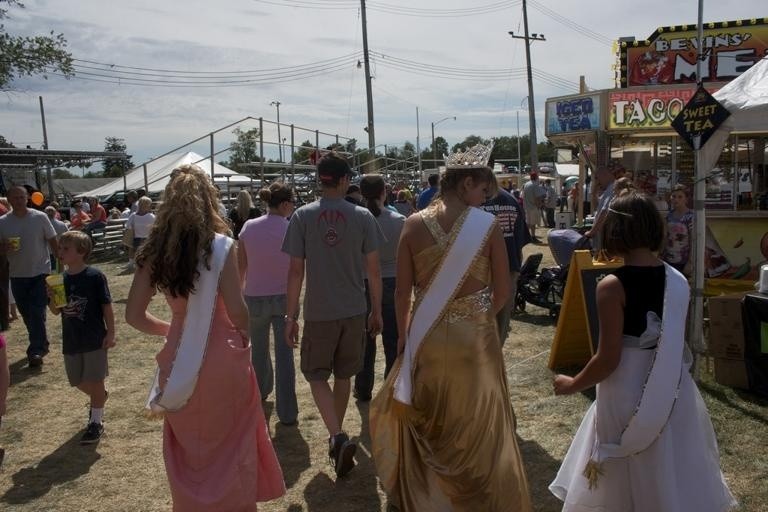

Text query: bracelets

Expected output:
[285,316,296,322]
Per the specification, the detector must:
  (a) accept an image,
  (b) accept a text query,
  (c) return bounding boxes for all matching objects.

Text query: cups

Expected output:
[44,274,67,309]
[758,264,768,293]
[8,237,21,252]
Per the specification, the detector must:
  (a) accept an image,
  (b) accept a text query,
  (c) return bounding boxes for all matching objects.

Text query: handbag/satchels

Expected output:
[122,229,134,249]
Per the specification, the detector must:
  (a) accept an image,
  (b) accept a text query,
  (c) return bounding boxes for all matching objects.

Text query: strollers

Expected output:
[514,228,594,321]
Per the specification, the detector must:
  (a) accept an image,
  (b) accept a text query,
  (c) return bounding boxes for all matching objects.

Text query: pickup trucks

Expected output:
[57,190,161,221]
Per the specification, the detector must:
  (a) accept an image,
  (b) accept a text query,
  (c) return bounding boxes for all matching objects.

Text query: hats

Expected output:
[319,154,357,182]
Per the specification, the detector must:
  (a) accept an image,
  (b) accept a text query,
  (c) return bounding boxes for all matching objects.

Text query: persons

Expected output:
[545,192,741,511]
[347,173,442,399]
[0,197,18,330]
[599,166,697,279]
[0,332,12,467]
[217,190,261,240]
[24,185,156,272]
[280,156,383,478]
[235,179,300,424]
[46,228,116,445]
[483,173,592,308]
[0,185,64,367]
[123,165,288,511]
[365,168,535,510]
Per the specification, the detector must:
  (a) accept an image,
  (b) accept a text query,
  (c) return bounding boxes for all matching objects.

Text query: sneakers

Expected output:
[29,353,43,367]
[329,431,357,477]
[79,390,108,444]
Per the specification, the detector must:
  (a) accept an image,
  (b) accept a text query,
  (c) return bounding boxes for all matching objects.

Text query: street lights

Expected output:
[282,138,286,162]
[270,101,282,162]
[432,117,458,166]
